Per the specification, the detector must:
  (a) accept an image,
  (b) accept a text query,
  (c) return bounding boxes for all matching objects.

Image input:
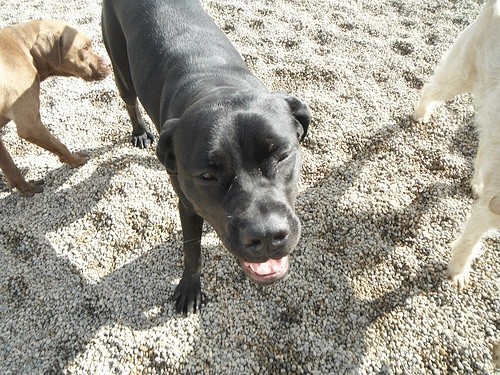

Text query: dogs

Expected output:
[101,1,310,318]
[0,21,111,196]
[413,1,499,284]
